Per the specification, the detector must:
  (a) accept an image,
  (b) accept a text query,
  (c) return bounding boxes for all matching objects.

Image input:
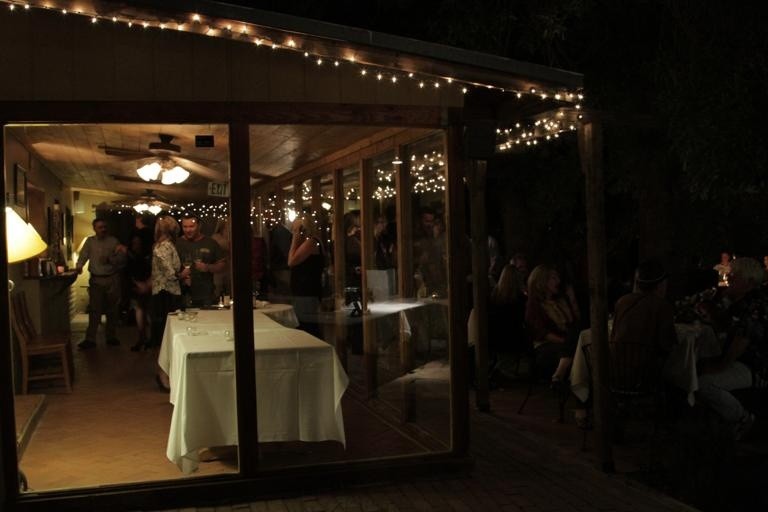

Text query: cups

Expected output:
[177,311,230,337]
[431,292,441,303]
[57,266,65,274]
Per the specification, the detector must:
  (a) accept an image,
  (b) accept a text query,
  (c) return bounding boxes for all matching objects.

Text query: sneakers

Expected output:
[104,337,122,346]
[76,339,98,349]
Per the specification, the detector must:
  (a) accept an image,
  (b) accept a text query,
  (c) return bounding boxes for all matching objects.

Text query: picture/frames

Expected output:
[13,163,29,208]
[26,181,48,258]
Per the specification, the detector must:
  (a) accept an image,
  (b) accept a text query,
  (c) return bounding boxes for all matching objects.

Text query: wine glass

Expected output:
[193,248,202,272]
[182,254,192,280]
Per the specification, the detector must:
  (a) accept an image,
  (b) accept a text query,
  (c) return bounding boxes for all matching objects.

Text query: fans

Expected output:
[111,188,185,212]
[97,133,220,165]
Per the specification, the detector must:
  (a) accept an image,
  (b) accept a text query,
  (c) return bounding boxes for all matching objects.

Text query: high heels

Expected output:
[155,373,169,393]
[130,338,148,352]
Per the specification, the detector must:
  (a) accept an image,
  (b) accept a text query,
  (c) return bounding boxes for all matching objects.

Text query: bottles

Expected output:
[344,287,361,306]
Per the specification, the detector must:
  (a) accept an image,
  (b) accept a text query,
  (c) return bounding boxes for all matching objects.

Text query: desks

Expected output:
[207,301,299,329]
[156,308,352,475]
[297,292,446,389]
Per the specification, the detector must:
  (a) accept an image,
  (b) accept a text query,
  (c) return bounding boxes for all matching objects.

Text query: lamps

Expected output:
[136,156,190,185]
[133,203,163,215]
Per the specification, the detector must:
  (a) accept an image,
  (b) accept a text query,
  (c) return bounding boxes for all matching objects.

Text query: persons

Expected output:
[76,218,125,349]
[112,206,768,442]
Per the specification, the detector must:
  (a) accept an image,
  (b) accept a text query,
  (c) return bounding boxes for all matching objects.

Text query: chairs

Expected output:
[598,341,676,475]
[519,315,577,416]
[9,290,72,395]
